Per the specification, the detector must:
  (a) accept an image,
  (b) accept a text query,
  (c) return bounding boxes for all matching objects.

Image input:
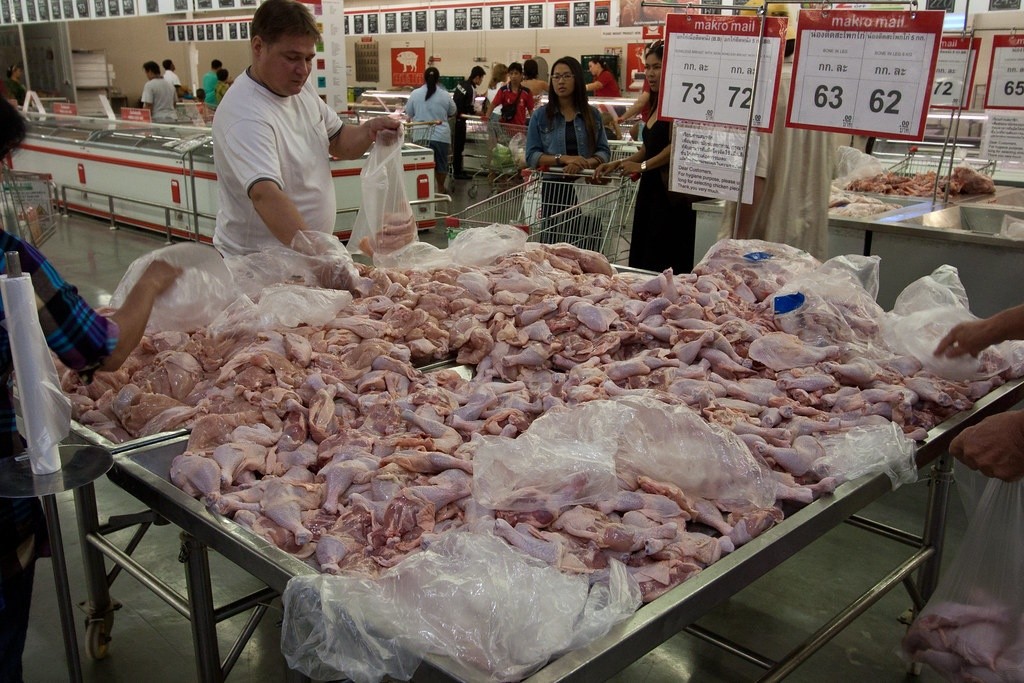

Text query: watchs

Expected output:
[641,161,646,172]
[555,154,561,165]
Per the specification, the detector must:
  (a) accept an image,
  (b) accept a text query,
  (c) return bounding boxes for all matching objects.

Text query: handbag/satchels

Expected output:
[573,175,621,218]
[500,86,523,120]
[346,122,418,254]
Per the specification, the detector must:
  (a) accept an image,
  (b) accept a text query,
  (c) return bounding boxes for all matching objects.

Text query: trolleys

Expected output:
[604,119,642,161]
[444,167,641,266]
[462,113,527,203]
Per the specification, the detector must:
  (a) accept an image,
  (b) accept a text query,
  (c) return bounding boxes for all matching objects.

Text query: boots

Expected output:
[453,156,473,179]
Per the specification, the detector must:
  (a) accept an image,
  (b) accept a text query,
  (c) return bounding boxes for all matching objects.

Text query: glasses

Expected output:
[551,72,575,82]
[650,40,663,48]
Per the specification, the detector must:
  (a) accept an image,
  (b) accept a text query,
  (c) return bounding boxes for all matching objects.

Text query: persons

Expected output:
[521,60,550,126]
[203,60,223,104]
[0,96,181,683]
[453,65,486,180]
[603,113,622,140]
[6,64,26,105]
[405,67,457,193]
[196,88,206,102]
[212,0,403,292]
[486,64,509,121]
[717,0,836,257]
[141,61,178,121]
[162,59,181,91]
[593,45,716,275]
[619,43,653,140]
[215,69,229,103]
[585,58,626,117]
[526,56,611,254]
[486,61,534,134]
[934,304,1024,483]
